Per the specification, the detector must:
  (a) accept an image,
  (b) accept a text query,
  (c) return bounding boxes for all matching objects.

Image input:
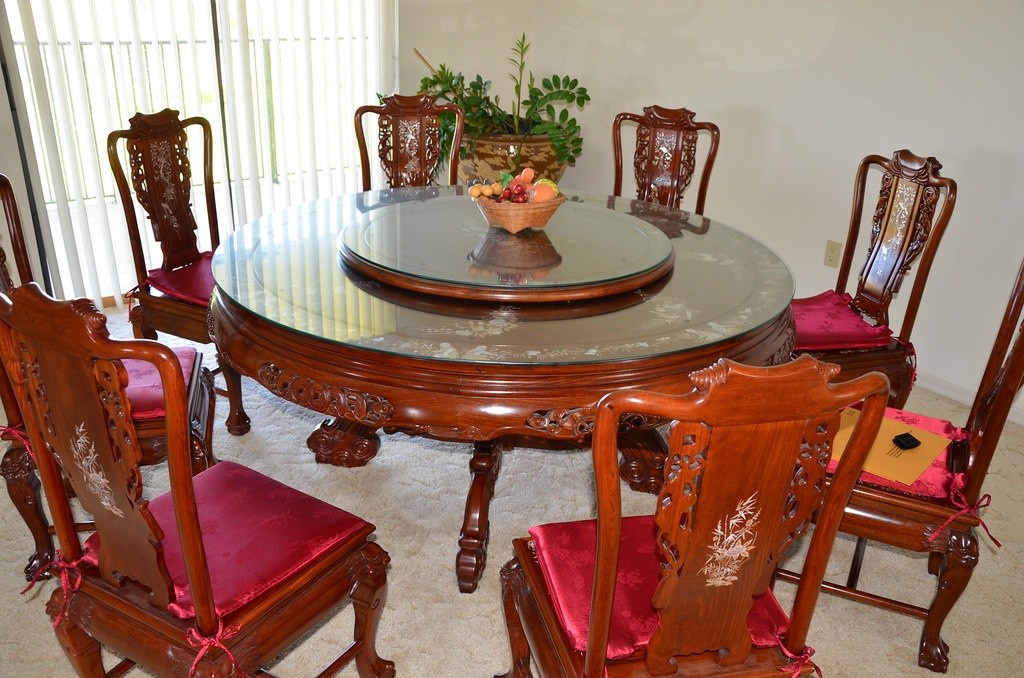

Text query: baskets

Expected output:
[475,190,565,234]
[467,226,562,273]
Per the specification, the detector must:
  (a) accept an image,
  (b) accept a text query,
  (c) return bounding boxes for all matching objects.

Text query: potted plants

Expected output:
[377,32,590,184]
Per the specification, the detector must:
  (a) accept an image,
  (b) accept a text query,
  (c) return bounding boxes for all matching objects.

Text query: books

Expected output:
[830,406,952,487]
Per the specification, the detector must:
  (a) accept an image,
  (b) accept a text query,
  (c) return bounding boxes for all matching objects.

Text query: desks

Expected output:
[206,186,797,593]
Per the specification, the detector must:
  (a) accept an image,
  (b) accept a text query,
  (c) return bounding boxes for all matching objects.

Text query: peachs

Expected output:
[526,183,554,204]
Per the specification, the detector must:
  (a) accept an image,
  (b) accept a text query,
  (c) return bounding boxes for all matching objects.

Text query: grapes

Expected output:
[497,184,528,203]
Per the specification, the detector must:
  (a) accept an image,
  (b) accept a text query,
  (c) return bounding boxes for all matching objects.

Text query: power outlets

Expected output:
[823,240,842,268]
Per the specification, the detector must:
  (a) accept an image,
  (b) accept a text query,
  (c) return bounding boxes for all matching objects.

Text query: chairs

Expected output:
[0,282,397,678]
[355,93,465,192]
[769,258,1024,673]
[107,108,251,435]
[0,173,224,583]
[493,356,889,678]
[789,150,957,410]
[613,105,720,217]
